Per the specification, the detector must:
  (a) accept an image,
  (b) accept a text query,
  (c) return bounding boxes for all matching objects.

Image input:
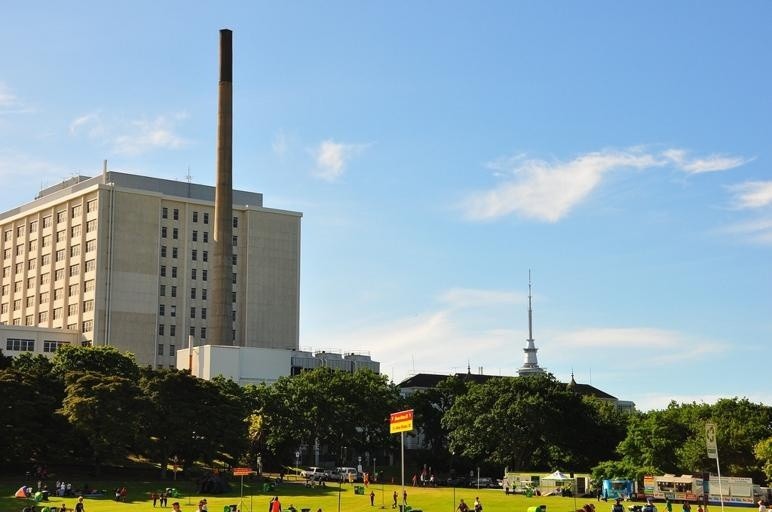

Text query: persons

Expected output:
[199,498,208,511]
[370,491,376,505]
[348,472,354,486]
[36,478,73,497]
[474,497,482,511]
[61,504,66,511]
[363,471,369,487]
[152,490,167,507]
[757,500,767,511]
[173,502,179,510]
[269,497,281,511]
[112,486,128,502]
[75,496,84,512]
[458,499,468,511]
[264,470,326,492]
[505,481,516,494]
[581,486,705,511]
[393,490,407,507]
[412,471,435,487]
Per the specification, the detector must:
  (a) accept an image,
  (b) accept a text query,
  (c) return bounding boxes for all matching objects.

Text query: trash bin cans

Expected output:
[526,489,532,497]
[263,482,276,493]
[166,488,177,497]
[354,486,364,494]
[399,505,412,512]
[535,505,546,512]
[224,505,237,512]
[35,491,48,501]
[41,507,56,512]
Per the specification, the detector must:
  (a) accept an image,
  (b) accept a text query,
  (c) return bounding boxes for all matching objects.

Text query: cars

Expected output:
[300,466,379,483]
[446,476,504,488]
